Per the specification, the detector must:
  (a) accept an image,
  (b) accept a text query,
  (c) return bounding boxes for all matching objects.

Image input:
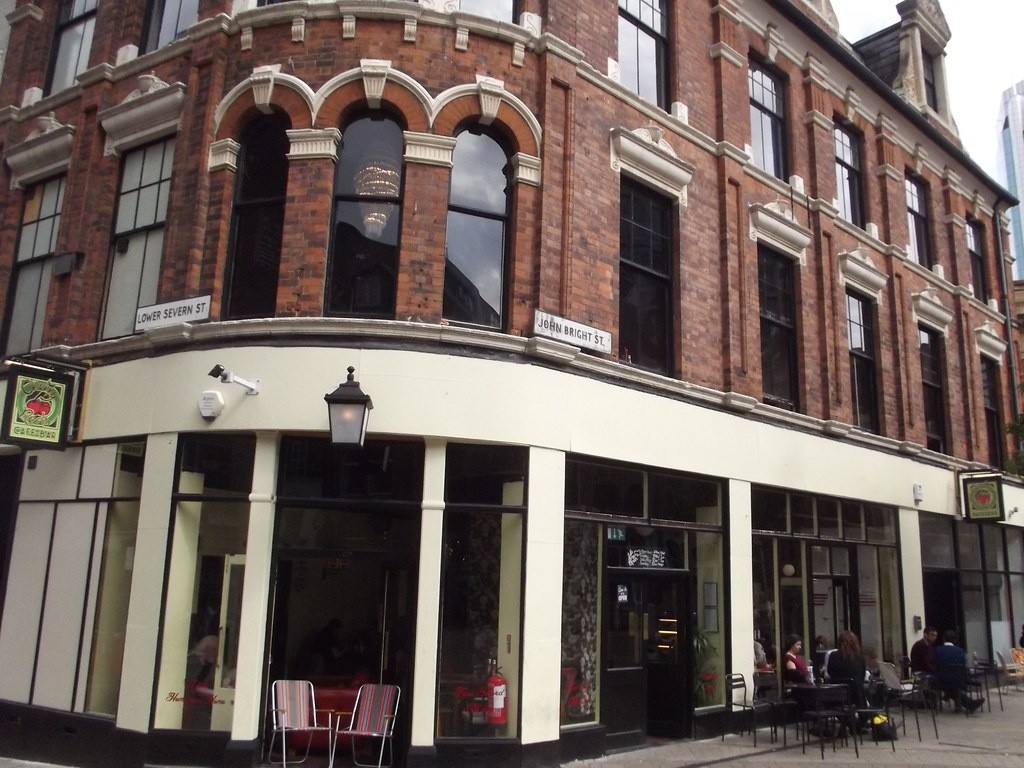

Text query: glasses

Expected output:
[931,633,938,638]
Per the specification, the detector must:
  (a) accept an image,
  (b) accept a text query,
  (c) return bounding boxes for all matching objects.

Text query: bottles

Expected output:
[973,652,978,666]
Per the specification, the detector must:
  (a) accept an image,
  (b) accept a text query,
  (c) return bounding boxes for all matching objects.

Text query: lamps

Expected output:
[324,366,374,448]
[353,139,401,237]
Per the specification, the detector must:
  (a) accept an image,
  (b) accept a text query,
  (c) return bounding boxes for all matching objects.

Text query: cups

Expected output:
[807,666,813,672]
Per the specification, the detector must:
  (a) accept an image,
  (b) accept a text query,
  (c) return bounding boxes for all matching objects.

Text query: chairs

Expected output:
[752,672,799,747]
[269,680,335,768]
[791,647,1024,759]
[722,673,773,747]
[331,683,400,768]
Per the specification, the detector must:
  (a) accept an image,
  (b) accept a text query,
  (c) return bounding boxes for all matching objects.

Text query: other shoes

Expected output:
[823,729,834,737]
[838,725,849,739]
[851,720,868,735]
[953,706,963,713]
[808,724,822,736]
[969,697,985,713]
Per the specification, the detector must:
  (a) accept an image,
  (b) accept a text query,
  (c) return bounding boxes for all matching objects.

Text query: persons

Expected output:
[312,618,350,667]
[753,624,986,739]
[187,633,235,688]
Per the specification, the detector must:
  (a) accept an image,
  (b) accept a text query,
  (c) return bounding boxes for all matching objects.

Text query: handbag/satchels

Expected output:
[871,714,894,738]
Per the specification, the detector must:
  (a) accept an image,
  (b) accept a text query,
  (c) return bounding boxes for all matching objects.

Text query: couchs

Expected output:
[289,656,374,752]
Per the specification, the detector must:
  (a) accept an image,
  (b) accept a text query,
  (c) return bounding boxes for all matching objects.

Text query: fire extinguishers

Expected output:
[488,668,508,725]
